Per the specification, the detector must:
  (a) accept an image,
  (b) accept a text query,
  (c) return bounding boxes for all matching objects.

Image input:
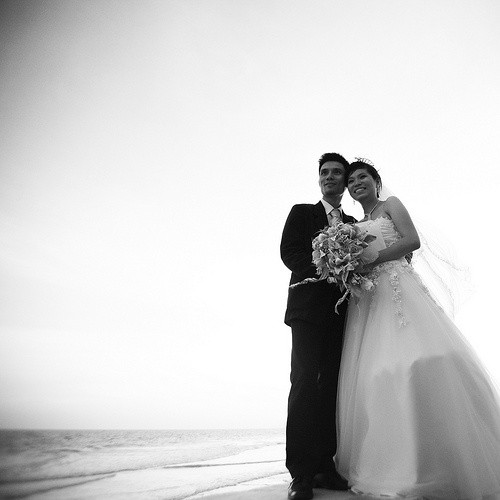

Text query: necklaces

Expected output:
[364,200,380,220]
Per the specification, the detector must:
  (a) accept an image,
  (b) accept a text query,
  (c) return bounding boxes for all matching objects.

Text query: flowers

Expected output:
[288,223,379,315]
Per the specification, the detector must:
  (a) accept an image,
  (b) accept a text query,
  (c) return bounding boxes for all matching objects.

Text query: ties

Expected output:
[330,209,341,227]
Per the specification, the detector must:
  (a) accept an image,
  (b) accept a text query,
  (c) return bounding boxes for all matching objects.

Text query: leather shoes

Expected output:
[313,470,351,490]
[288,476,314,500]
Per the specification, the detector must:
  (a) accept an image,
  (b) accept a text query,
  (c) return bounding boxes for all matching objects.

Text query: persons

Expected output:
[332,157,500,500]
[281,153,358,500]
